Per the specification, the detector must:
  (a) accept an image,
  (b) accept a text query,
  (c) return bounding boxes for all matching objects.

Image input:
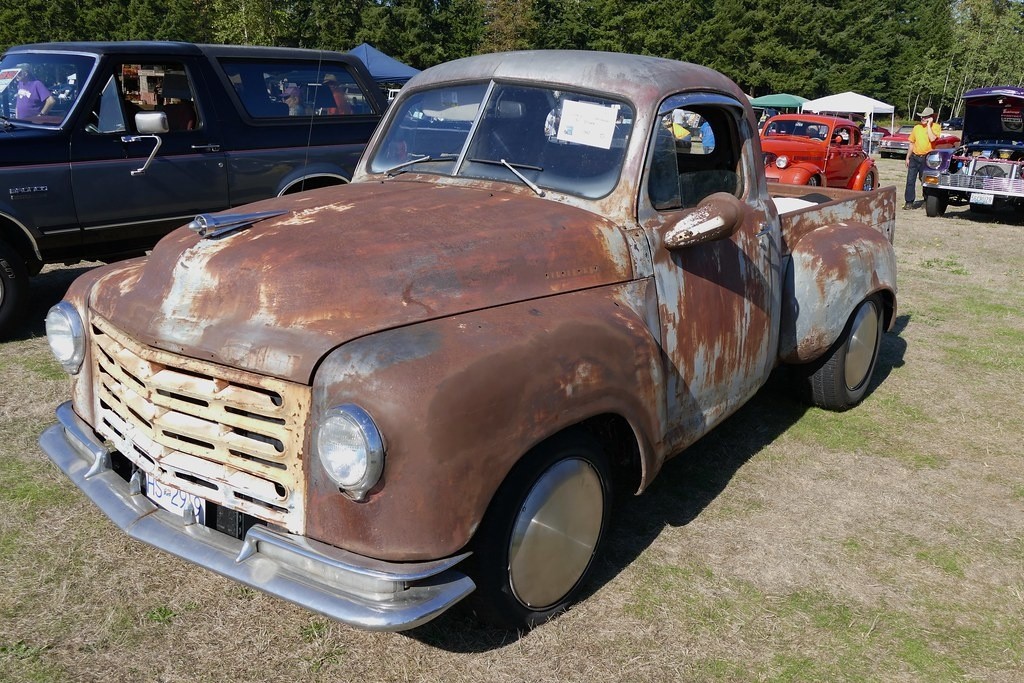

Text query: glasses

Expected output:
[921,116,930,119]
[17,73,27,77]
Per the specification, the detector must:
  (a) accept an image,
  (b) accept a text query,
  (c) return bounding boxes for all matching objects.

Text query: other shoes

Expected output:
[921,199,928,208]
[902,202,913,210]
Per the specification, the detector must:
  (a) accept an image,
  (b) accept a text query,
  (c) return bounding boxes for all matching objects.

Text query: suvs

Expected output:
[917,85,1024,218]
[0,40,392,343]
[755,114,880,194]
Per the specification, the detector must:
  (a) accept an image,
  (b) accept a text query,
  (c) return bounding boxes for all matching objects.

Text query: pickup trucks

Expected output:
[39,47,901,634]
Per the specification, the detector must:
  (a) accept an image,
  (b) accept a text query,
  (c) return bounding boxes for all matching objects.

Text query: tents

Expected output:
[747,93,809,114]
[284,43,421,84]
[801,91,894,155]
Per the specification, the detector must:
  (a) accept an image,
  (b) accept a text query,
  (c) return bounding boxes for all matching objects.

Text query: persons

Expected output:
[279,87,306,117]
[699,121,715,154]
[15,64,56,118]
[324,74,352,114]
[672,109,685,125]
[665,122,690,142]
[903,108,940,210]
[768,107,777,117]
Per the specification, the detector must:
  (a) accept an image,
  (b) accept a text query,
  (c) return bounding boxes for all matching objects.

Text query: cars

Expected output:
[860,115,966,161]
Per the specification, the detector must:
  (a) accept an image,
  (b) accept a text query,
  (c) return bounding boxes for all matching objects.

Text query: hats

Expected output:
[279,86,299,97]
[322,73,338,83]
[16,63,30,71]
[917,107,934,116]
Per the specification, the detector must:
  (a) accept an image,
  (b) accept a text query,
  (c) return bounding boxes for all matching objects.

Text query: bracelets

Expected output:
[40,112,43,116]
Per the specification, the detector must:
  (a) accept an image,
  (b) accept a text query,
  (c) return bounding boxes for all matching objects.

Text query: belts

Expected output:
[912,153,926,158]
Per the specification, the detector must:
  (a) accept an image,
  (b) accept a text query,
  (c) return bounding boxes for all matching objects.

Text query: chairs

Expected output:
[161,75,196,132]
[680,170,743,210]
